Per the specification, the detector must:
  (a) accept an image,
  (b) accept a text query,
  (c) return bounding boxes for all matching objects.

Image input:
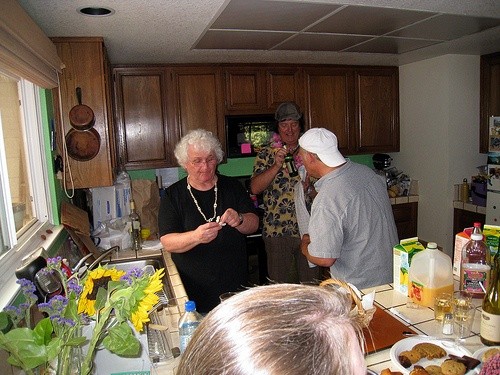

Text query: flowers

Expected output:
[0,256,143,375]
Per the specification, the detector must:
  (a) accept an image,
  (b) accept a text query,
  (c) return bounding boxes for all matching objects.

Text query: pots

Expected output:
[65,87,100,162]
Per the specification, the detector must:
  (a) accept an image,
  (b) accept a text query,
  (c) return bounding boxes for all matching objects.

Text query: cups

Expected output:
[434,292,452,322]
[453,290,473,319]
[453,304,476,338]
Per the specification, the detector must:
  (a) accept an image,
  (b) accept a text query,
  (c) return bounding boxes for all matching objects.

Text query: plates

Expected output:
[390,336,473,375]
[473,346,500,373]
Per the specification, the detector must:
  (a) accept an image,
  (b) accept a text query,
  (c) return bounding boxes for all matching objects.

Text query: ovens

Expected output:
[246,227,268,288]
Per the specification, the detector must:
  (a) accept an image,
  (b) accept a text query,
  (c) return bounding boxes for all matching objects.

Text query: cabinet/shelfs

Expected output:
[48,36,119,190]
[392,202,419,239]
[303,66,400,150]
[113,63,227,170]
[225,64,305,113]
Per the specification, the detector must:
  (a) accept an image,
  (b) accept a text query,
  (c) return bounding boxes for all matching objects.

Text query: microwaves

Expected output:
[226,114,277,157]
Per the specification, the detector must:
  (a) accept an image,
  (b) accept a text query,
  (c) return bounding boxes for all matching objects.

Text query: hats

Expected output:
[299,128,346,167]
[275,102,302,120]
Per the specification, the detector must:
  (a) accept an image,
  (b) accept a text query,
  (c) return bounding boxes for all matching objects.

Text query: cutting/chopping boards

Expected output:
[360,304,419,354]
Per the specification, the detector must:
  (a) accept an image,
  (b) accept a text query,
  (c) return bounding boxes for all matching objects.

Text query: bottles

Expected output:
[178,301,204,355]
[480,236,500,346]
[127,200,143,251]
[281,141,298,177]
[407,240,454,307]
[472,221,482,235]
[443,314,453,336]
[462,179,469,203]
[460,235,491,299]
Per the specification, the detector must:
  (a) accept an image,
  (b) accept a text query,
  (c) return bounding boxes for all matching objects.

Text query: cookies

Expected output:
[399,342,500,375]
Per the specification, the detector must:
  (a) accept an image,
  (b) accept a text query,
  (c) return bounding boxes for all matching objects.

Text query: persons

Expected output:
[298,127,400,291]
[251,103,319,288]
[176,283,367,375]
[157,128,260,314]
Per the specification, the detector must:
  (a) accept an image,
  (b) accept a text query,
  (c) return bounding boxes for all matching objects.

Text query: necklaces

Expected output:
[187,175,218,222]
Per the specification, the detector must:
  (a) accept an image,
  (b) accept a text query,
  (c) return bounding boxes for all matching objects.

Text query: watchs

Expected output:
[237,210,243,225]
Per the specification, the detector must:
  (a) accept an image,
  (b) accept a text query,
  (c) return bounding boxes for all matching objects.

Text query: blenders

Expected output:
[373,154,393,184]
